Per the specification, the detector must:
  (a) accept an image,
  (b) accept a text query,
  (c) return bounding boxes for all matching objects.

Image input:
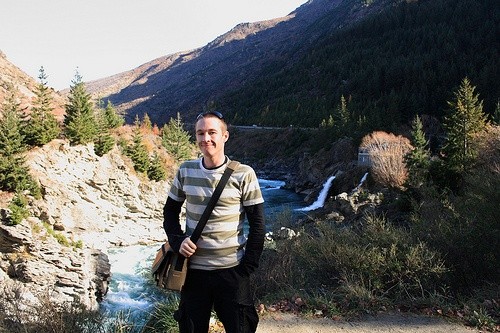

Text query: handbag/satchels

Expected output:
[151,241,188,293]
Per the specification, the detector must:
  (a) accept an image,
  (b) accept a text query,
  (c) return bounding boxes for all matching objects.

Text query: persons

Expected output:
[163,111,266,333]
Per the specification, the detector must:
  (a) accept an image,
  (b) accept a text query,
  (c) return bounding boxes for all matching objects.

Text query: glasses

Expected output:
[195,111,225,122]
